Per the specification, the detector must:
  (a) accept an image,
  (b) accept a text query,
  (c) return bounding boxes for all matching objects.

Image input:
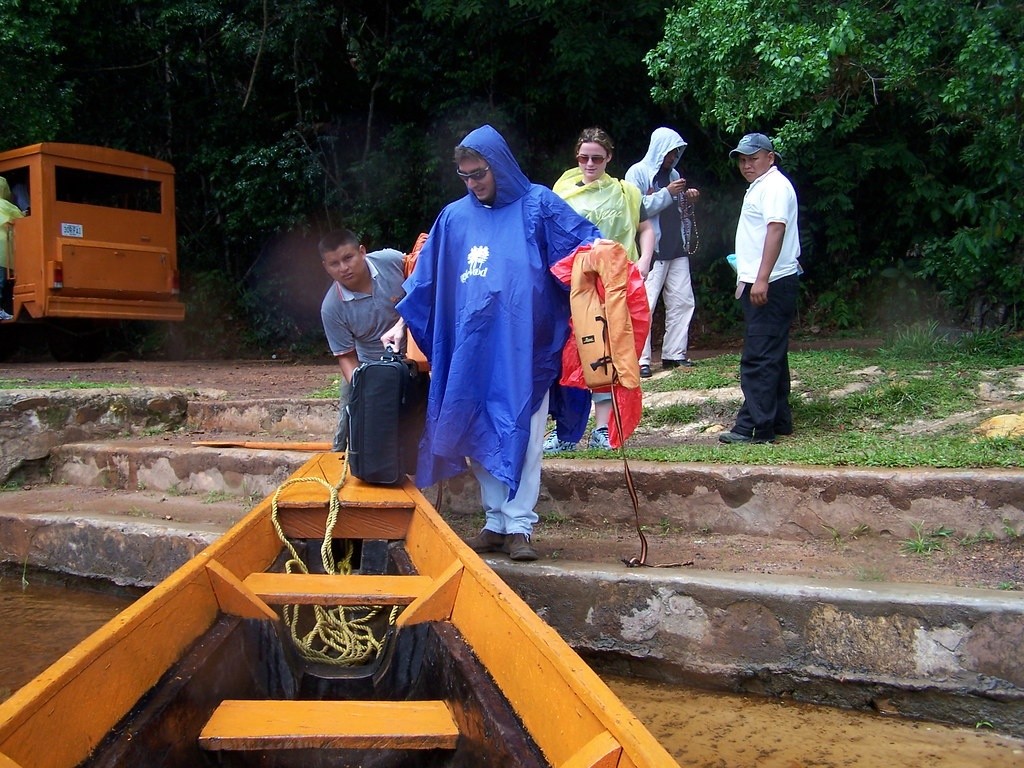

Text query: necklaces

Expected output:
[680,192,699,255]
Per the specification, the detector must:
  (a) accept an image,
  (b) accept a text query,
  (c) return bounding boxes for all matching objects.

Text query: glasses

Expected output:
[457,166,489,181]
[576,155,607,164]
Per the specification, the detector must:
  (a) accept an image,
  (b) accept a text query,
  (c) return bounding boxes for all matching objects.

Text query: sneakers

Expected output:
[588,426,617,452]
[544,438,577,454]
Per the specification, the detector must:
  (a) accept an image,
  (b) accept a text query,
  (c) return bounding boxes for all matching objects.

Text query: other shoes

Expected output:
[719,433,775,444]
[504,533,539,560]
[463,530,504,553]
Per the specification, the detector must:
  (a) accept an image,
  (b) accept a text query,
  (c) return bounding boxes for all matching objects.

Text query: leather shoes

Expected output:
[639,364,652,377]
[662,359,697,368]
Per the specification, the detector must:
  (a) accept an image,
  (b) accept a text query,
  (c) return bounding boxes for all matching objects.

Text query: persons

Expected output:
[380,124,602,557]
[320,126,802,459]
[0,177,25,320]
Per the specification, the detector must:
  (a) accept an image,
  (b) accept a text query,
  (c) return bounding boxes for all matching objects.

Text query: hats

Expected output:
[729,133,772,159]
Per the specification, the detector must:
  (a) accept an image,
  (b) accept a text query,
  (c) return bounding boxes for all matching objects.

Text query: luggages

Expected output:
[346,347,409,486]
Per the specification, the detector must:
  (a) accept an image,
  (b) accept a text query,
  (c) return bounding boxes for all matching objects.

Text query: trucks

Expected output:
[0,141,186,360]
